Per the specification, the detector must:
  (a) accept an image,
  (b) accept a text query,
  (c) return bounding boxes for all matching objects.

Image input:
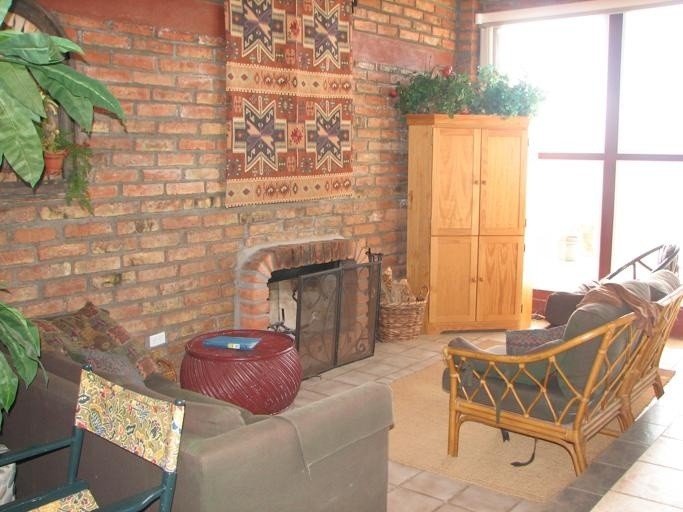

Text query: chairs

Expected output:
[0,365,186,511]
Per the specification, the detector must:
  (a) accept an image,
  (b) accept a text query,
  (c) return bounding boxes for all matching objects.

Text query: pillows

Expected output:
[26,300,156,381]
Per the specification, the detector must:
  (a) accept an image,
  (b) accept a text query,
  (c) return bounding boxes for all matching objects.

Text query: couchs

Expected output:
[1,311,393,511]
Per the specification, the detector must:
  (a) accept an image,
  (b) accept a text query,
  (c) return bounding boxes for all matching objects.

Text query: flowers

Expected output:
[388,64,544,124]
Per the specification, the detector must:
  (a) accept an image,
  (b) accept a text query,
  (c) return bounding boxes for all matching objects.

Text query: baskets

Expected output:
[376,285,429,342]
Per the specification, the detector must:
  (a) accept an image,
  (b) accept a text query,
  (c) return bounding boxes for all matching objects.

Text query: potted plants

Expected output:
[41,128,72,173]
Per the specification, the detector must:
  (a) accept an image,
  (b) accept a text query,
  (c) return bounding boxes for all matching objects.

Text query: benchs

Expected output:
[441,245,683,479]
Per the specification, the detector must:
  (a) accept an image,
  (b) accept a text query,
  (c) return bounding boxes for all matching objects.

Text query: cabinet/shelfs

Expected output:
[403,115,530,337]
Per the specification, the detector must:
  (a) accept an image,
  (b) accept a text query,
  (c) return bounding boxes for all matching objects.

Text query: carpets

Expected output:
[388,340,676,507]
